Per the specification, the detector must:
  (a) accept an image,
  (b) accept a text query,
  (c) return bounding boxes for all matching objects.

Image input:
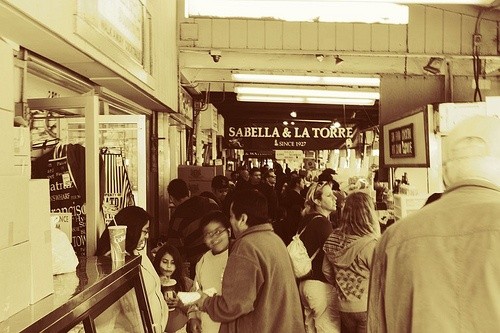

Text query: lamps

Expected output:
[330,118,342,130]
[334,54,344,65]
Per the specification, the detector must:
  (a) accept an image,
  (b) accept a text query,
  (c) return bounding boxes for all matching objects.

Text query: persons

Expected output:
[94,206,169,333]
[367,115,500,333]
[152,163,383,333]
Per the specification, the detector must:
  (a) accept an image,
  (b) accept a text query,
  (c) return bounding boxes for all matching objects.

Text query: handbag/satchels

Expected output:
[54,271,80,308]
[51,217,79,275]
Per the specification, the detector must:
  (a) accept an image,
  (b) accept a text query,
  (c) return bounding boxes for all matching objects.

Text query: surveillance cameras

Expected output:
[209,51,221,62]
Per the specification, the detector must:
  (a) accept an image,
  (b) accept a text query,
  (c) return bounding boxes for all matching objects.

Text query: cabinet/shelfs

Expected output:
[0,255,157,333]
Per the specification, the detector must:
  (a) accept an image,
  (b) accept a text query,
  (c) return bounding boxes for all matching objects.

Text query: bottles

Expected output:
[393,182,399,193]
[402,172,408,184]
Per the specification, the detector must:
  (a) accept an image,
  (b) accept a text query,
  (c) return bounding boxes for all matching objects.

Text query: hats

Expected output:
[212,175,231,188]
[443,114,500,162]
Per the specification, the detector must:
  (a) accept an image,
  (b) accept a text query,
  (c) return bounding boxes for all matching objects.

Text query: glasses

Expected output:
[142,228,149,234]
[269,175,277,178]
[203,229,226,238]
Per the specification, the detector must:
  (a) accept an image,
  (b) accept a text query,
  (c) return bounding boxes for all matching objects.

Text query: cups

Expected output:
[162,279,177,311]
[108,225,127,261]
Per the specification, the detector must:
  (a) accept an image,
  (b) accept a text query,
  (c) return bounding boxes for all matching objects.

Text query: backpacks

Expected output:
[286,214,326,278]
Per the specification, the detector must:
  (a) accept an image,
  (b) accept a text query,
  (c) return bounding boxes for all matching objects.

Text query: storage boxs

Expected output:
[177,164,224,196]
[0,241,31,324]
[0,37,16,112]
[14,126,31,178]
[0,177,29,250]
[0,109,15,176]
[30,240,55,304]
[29,179,51,241]
